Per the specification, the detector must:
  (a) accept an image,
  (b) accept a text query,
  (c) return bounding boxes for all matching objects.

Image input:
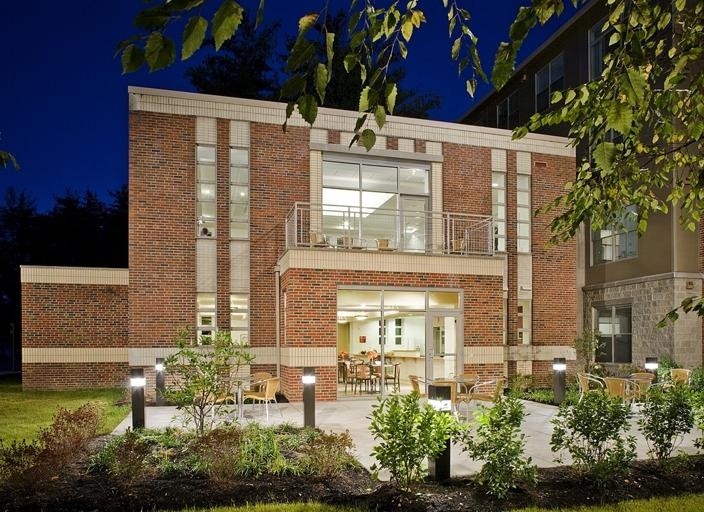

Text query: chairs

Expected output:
[408,373,509,425]
[337,350,400,395]
[310,232,398,252]
[211,372,284,426]
[576,368,692,414]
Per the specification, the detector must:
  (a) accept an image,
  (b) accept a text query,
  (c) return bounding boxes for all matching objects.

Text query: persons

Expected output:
[199,228,209,237]
